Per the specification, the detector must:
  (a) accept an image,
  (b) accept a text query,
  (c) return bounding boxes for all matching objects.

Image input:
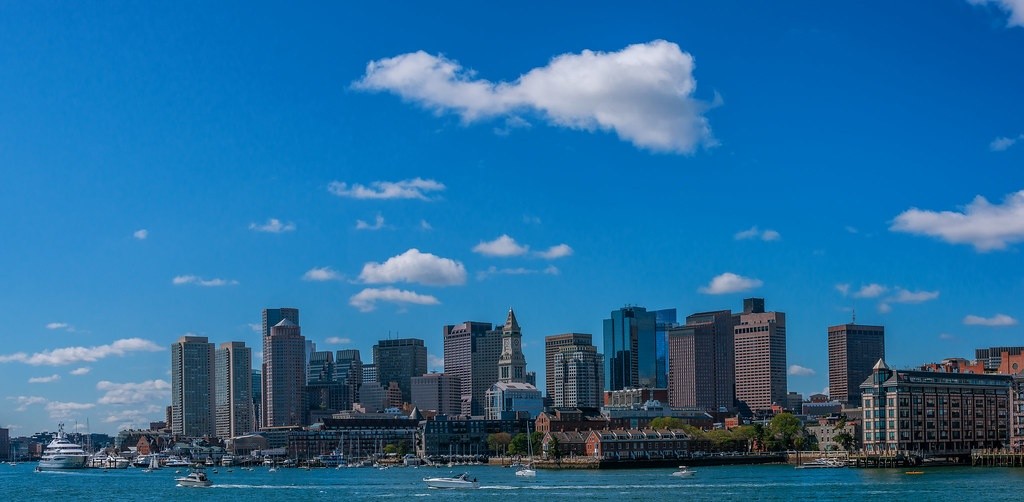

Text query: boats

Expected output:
[803,458,845,468]
[38,420,91,469]
[423,473,481,490]
[174,466,213,487]
[35,469,42,472]
[671,470,697,477]
[205,456,215,467]
[167,446,196,466]
[904,471,924,474]
[90,452,130,469]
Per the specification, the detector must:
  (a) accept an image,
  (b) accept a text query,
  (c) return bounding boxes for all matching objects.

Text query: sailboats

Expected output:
[335,433,384,469]
[516,420,537,478]
[147,451,163,470]
[10,446,17,466]
[447,444,483,465]
[228,440,311,472]
